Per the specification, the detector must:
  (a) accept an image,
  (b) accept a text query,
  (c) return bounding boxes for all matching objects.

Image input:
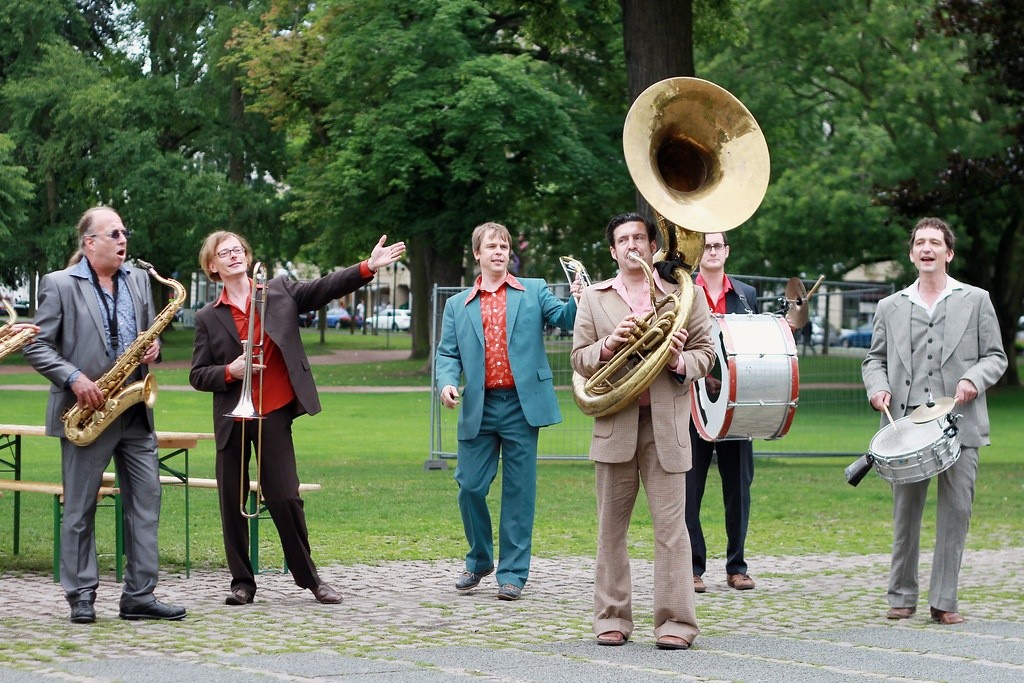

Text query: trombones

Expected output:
[220,260,268,518]
[558,255,593,309]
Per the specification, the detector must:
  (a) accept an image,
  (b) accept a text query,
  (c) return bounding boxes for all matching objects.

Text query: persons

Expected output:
[436,223,580,600]
[190,232,405,604]
[802,320,816,357]
[571,213,716,649]
[684,233,799,591]
[339,299,392,332]
[0,324,40,356]
[24,207,186,623]
[862,218,1008,624]
[13,295,22,305]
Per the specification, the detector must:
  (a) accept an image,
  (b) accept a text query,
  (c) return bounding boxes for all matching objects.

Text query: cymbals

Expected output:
[910,396,956,425]
[784,275,810,330]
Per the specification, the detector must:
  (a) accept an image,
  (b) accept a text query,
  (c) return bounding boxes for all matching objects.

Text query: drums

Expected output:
[868,415,964,486]
[691,312,802,444]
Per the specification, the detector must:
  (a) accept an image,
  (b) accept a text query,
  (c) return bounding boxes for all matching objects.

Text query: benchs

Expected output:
[102,472,321,574]
[1,479,120,582]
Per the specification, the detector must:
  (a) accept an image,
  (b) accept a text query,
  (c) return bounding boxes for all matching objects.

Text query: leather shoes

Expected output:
[597,631,625,645]
[456,564,494,589]
[694,574,706,591]
[71,601,95,623]
[656,636,688,649]
[498,584,521,599]
[120,599,186,620]
[225,589,250,604]
[931,606,964,624]
[727,574,755,590]
[309,580,344,604]
[888,605,917,618]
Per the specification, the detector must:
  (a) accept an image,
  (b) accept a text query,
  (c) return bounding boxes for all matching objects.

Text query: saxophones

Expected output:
[0,296,38,360]
[59,258,188,447]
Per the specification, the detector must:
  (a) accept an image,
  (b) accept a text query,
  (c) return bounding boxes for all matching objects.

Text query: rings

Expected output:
[677,346,684,349]
[442,401,444,405]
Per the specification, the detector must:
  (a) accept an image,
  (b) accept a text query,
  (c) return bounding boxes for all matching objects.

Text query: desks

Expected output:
[0,424,215,582]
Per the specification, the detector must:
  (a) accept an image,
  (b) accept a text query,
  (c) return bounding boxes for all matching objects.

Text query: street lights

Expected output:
[386,261,406,332]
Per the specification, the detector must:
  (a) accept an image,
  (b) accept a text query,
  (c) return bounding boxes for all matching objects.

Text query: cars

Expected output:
[0,301,30,316]
[366,308,412,332]
[1014,315,1024,351]
[311,307,365,331]
[794,321,875,348]
[298,308,317,327]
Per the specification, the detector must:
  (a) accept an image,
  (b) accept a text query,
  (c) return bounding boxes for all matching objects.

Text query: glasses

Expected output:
[213,245,245,258]
[703,243,725,251]
[91,229,130,240]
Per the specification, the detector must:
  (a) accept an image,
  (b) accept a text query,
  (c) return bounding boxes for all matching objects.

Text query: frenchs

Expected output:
[568,76,772,420]
